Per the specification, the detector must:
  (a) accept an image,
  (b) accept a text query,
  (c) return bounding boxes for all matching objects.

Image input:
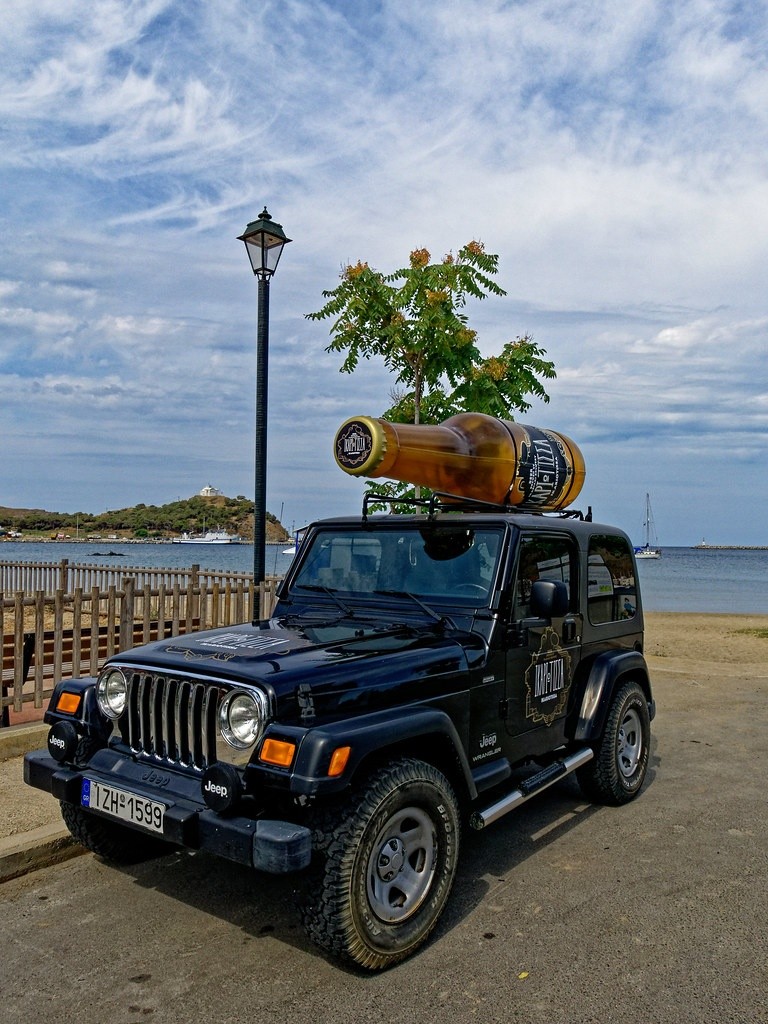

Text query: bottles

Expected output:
[332,412,585,514]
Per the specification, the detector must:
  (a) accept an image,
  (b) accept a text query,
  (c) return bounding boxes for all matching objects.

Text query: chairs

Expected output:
[400,559,474,596]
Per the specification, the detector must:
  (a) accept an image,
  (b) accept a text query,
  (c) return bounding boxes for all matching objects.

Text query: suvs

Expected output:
[25,412,656,975]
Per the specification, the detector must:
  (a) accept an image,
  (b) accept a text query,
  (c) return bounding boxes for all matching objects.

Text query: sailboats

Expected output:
[632,492,663,559]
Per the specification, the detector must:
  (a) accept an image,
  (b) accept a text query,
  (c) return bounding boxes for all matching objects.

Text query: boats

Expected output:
[168,529,240,545]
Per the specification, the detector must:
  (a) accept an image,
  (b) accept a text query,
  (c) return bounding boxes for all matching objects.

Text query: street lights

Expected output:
[237,202,294,626]
